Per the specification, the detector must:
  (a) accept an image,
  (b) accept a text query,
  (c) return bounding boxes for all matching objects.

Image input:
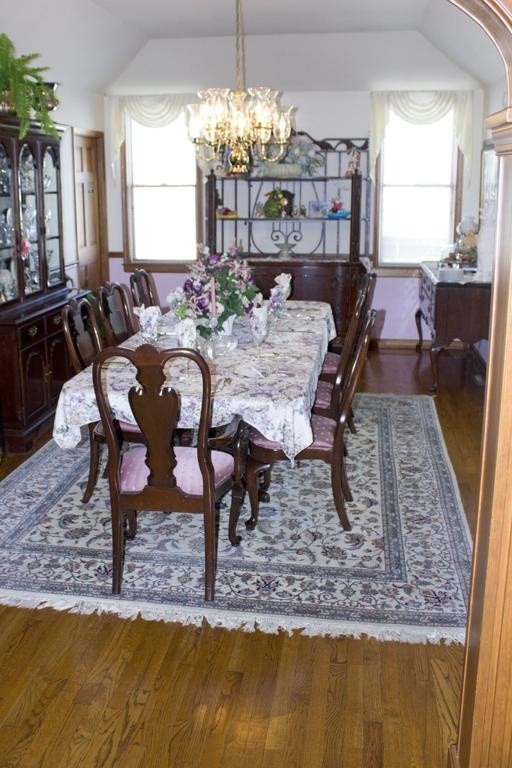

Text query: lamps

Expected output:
[185,0,296,174]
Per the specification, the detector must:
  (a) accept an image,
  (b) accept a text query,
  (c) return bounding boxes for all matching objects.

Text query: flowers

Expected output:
[183,242,259,338]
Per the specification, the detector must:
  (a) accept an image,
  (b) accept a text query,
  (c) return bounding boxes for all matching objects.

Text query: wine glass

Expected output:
[140,323,157,352]
[268,301,286,339]
[171,298,184,327]
[250,320,269,360]
[177,333,198,382]
[278,286,291,314]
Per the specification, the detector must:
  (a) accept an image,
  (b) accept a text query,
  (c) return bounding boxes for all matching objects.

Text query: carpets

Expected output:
[1,393,474,646]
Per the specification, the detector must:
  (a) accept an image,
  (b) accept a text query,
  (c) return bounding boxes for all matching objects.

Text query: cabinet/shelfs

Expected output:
[205,125,373,348]
[0,116,94,453]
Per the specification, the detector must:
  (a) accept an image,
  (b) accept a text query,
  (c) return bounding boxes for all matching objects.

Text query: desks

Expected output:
[414,261,491,392]
